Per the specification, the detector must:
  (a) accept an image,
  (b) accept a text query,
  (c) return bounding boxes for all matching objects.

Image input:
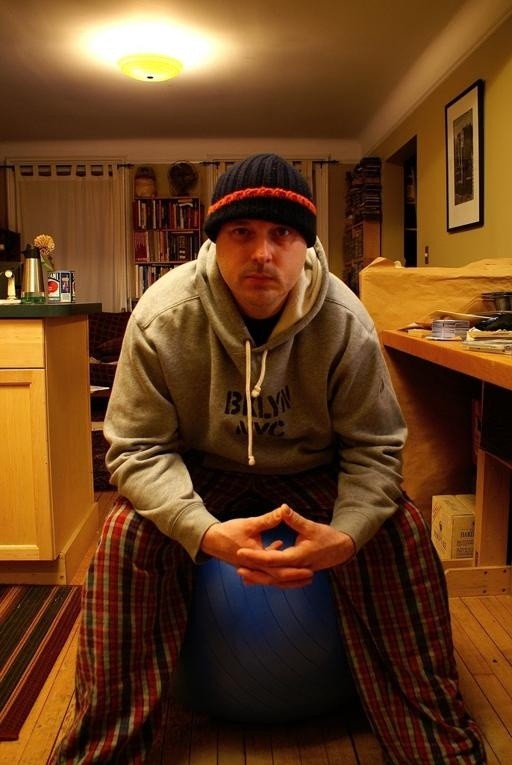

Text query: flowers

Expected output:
[33,234,57,273]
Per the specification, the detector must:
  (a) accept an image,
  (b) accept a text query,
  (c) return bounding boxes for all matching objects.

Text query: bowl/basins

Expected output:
[479,291,511,312]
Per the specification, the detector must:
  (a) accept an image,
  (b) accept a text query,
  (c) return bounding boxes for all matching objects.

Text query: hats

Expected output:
[202,154,316,247]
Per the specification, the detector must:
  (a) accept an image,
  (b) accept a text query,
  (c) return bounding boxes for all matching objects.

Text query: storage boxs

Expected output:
[430,494,476,561]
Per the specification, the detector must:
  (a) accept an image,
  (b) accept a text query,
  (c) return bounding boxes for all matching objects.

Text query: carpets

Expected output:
[2,585,83,741]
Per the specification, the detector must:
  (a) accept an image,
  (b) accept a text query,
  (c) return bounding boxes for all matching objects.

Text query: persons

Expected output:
[42,151,485,763]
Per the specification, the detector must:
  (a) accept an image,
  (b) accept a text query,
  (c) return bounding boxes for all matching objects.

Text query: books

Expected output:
[133,198,200,300]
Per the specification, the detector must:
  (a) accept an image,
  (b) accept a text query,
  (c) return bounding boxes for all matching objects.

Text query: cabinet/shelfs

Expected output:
[342,219,380,295]
[132,197,205,309]
[0,315,98,585]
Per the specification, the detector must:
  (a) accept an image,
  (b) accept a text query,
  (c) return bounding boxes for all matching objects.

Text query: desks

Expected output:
[383,329,512,595]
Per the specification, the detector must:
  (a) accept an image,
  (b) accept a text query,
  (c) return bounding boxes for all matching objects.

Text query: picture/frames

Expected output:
[445,79,484,232]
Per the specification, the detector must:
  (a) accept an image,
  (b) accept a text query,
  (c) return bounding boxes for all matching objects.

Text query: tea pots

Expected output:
[20,244,47,304]
